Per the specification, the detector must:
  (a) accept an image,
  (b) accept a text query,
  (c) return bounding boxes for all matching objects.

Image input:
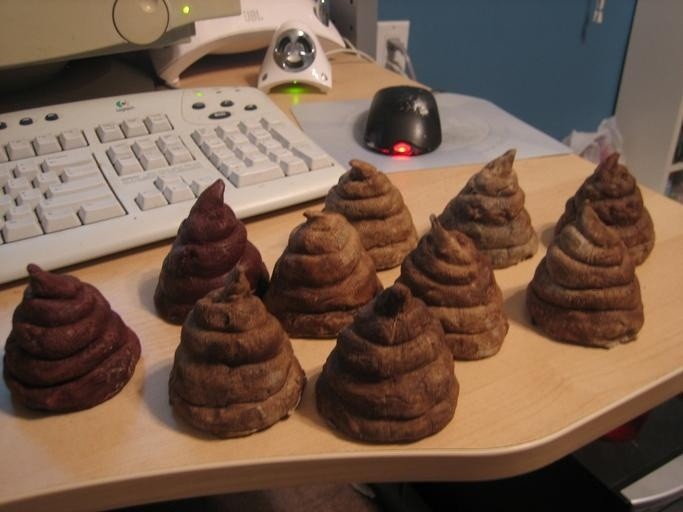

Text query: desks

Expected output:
[0,50,683,512]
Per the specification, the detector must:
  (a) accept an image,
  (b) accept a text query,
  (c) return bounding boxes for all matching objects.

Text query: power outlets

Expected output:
[376,21,411,74]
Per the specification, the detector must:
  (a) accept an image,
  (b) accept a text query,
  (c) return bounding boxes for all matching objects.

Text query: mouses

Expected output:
[364,84,442,157]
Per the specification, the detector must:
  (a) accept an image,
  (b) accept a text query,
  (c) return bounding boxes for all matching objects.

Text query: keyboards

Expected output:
[0,83,347,285]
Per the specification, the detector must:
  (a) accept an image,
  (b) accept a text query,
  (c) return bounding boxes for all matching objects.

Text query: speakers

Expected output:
[256,20,333,94]
